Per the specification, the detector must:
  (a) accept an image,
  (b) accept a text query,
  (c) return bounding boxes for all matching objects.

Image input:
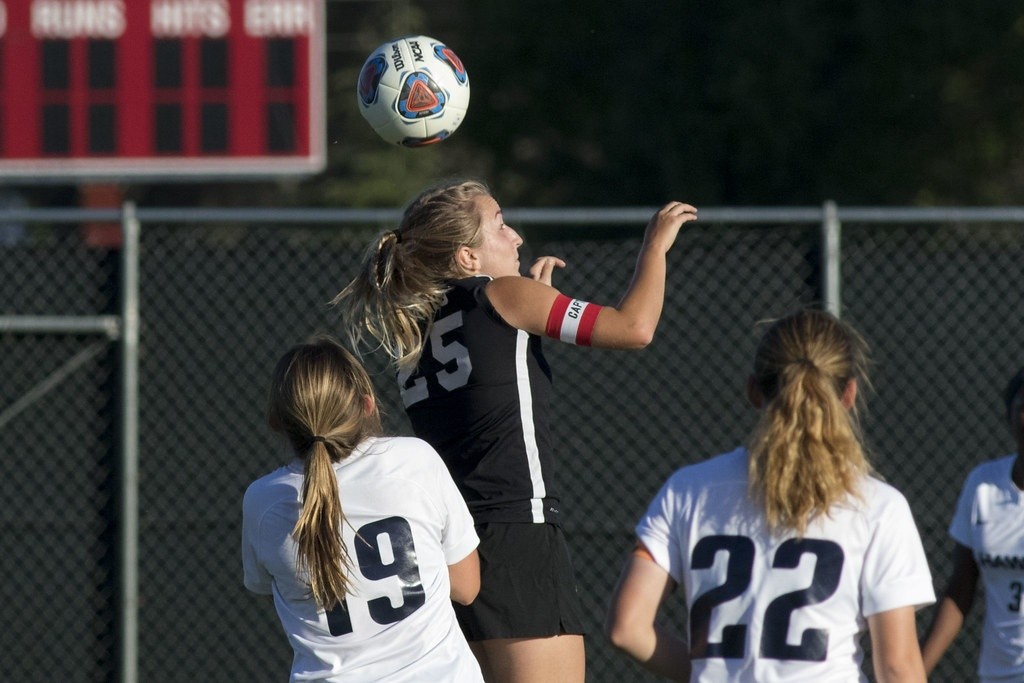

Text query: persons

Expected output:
[920,369,1024,683]
[236,338,493,683]
[329,181,697,683]
[605,306,937,683]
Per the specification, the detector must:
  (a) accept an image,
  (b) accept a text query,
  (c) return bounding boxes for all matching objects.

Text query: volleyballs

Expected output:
[356,34,471,150]
